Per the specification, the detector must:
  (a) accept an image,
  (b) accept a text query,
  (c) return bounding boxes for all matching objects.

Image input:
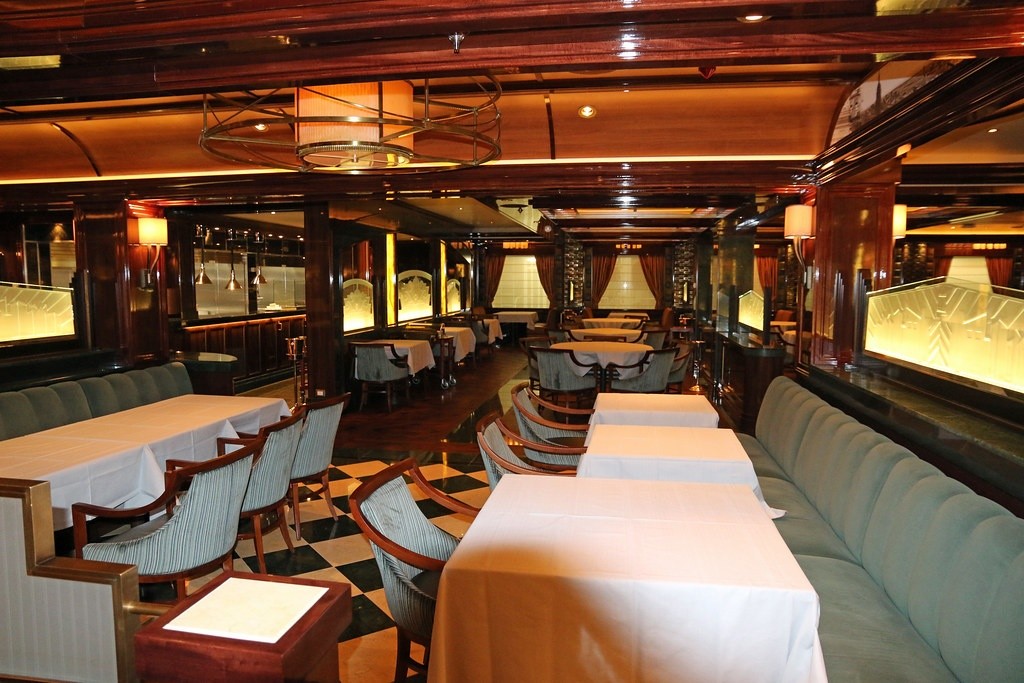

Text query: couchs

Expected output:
[0,362,195,440]
[731,375,1023,681]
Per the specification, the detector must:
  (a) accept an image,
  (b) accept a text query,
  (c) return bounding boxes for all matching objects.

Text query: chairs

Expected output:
[348,457,483,681]
[475,408,588,492]
[512,380,595,447]
[70,437,264,604]
[236,392,351,540]
[347,303,811,413]
[165,406,305,573]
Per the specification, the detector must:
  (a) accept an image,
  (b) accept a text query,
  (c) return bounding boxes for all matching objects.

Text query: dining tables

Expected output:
[476,317,502,344]
[783,329,796,354]
[581,317,644,329]
[607,312,648,319]
[444,473,819,682]
[551,341,655,393]
[443,326,477,361]
[585,422,751,483]
[593,392,719,428]
[770,319,797,334]
[367,339,429,406]
[0,394,284,531]
[570,327,648,343]
[494,311,539,347]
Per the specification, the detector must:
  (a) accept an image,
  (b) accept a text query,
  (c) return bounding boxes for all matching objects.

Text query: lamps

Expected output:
[226,241,242,290]
[295,78,414,168]
[253,243,267,284]
[785,205,817,272]
[195,237,212,283]
[138,217,167,289]
